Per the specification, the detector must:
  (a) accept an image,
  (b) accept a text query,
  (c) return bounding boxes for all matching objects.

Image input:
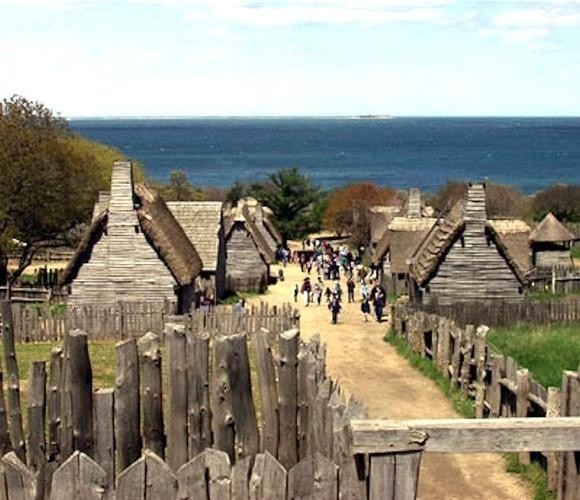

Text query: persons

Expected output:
[275,237,386,324]
[191,288,215,314]
[233,295,245,313]
